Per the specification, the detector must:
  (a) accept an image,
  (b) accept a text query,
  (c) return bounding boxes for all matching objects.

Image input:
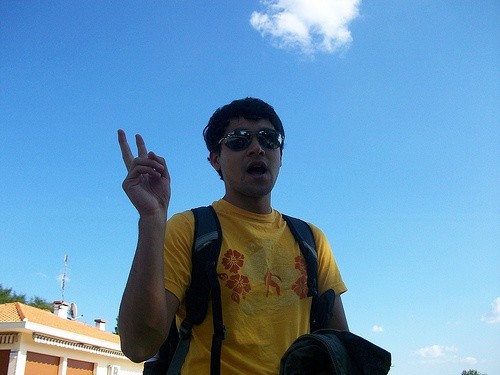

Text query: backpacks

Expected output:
[142,205,336,374]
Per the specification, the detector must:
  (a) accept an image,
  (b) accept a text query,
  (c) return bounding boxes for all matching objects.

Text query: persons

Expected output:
[117,97,350,375]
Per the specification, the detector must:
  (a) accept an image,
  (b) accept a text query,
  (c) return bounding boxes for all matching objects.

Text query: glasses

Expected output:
[218,128,285,152]
